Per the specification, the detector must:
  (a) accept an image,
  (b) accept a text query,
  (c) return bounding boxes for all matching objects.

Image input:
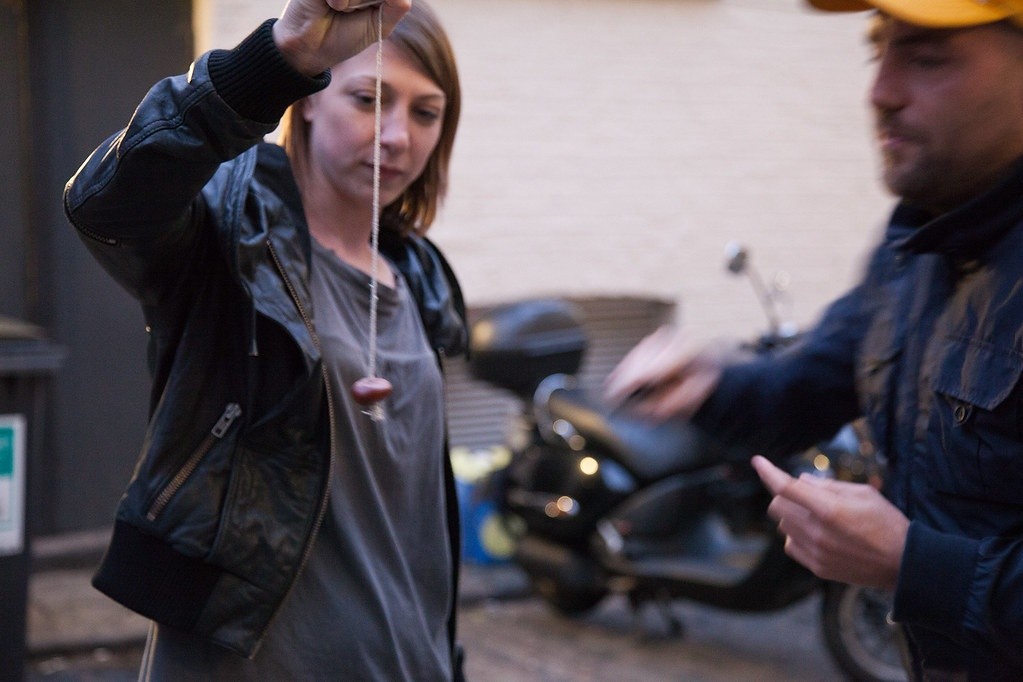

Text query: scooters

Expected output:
[463,244,913,682]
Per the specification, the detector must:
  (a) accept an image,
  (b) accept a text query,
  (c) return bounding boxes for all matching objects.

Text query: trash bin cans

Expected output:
[0,319,70,681]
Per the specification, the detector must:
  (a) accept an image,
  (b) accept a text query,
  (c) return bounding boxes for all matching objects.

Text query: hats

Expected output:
[800,0,1023,22]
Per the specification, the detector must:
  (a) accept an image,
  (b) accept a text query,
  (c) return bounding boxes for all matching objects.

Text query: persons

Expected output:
[61,1,488,682]
[592,1,1023,682]
